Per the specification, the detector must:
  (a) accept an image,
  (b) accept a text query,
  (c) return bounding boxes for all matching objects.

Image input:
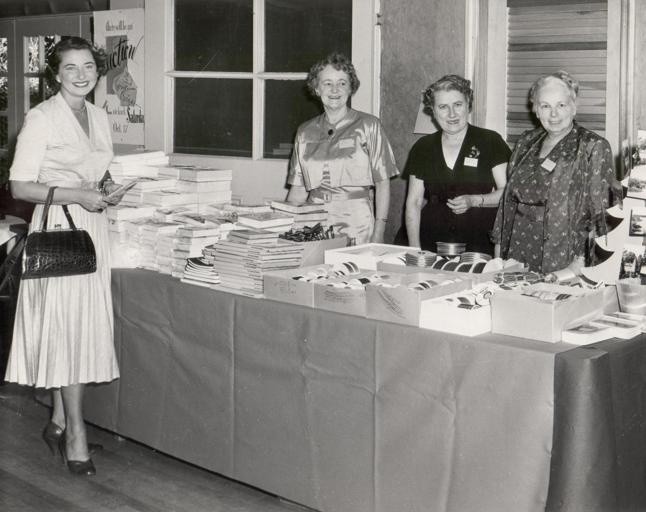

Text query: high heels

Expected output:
[59,429,96,476]
[44,422,103,464]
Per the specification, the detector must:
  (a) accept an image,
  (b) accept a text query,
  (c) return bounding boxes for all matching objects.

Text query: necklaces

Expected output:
[74,106,85,114]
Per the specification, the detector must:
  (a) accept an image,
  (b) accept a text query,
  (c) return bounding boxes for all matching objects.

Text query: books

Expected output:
[104,148,331,298]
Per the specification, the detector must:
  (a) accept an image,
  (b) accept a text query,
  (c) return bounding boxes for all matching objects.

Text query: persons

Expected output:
[490,70,612,282]
[286,52,400,244]
[394,75,513,250]
[8,36,124,478]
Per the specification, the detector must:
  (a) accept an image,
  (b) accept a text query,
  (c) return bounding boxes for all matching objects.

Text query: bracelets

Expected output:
[479,194,484,208]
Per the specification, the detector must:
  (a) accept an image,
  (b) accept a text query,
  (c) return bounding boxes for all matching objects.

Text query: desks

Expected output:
[34,264,646,511]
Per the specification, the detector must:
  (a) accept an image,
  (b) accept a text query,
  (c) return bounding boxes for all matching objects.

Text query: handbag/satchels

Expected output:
[21,230,96,280]
